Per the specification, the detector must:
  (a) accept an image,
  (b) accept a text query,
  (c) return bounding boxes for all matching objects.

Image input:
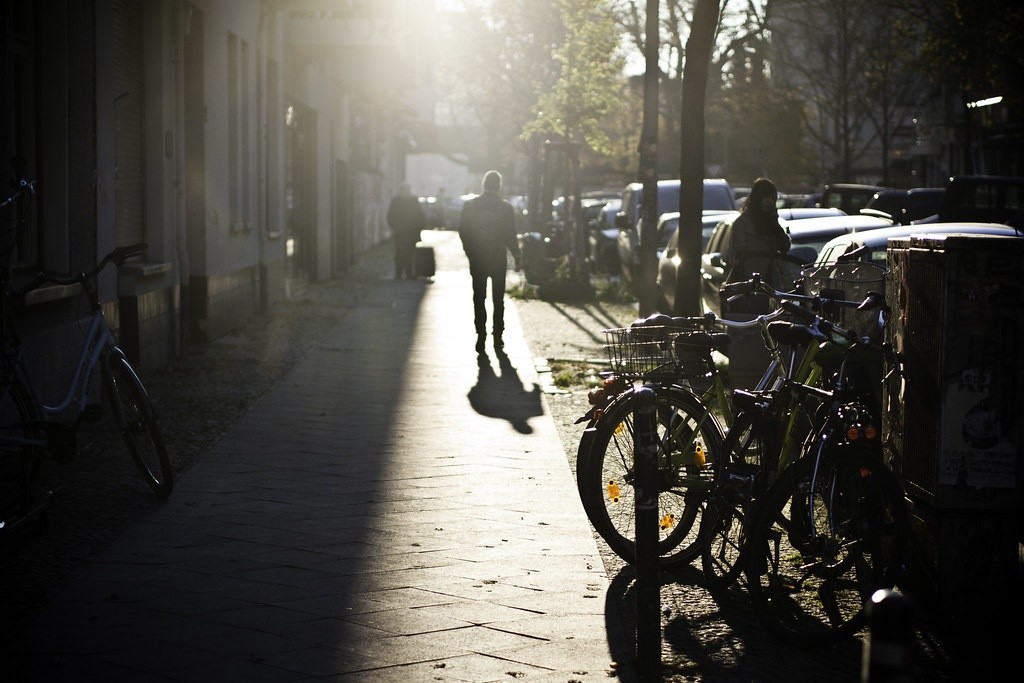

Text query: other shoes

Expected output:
[494,339,504,348]
[395,274,415,281]
[476,336,486,353]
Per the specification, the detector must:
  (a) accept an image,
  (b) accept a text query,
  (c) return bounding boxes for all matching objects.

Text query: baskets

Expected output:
[600,325,708,380]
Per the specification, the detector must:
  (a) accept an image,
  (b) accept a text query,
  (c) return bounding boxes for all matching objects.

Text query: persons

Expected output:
[459,173,522,351]
[388,183,424,280]
[721,178,791,413]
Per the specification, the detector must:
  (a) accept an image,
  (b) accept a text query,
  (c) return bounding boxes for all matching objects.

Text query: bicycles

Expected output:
[575,254,911,648]
[0,241,174,556]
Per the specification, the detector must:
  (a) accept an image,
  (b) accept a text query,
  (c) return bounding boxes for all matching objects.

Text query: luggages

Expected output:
[416,247,435,283]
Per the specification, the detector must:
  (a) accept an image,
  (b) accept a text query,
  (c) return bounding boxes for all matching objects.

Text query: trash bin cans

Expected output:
[720,259,803,430]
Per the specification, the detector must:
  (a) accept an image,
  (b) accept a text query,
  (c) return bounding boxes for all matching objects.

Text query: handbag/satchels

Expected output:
[719,280,769,321]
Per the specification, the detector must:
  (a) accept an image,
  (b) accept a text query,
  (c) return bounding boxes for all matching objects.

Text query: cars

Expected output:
[580,199,608,227]
[730,186,753,199]
[701,207,903,401]
[657,210,741,252]
[805,222,1024,333]
[587,199,623,274]
[552,195,577,228]
[777,173,1024,229]
[614,178,738,301]
[657,213,737,317]
[509,197,529,217]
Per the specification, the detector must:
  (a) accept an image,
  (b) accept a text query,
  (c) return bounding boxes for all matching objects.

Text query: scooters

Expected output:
[514,233,589,300]
